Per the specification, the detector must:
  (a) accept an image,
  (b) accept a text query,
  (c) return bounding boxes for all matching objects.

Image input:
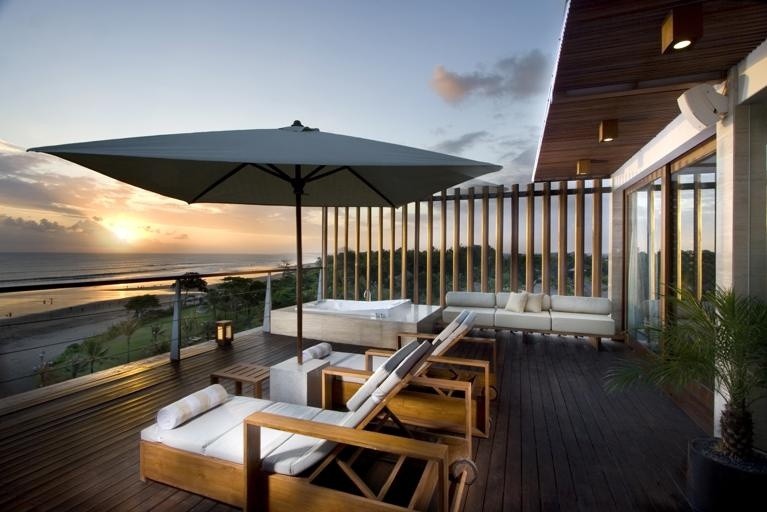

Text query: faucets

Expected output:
[363,289,371,302]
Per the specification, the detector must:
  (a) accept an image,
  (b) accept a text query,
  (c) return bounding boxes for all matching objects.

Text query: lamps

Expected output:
[573,158,592,176]
[655,3,708,59]
[595,116,619,145]
[214,317,236,350]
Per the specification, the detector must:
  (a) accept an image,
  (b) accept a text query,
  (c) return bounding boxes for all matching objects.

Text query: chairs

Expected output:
[296,308,498,440]
[133,336,502,512]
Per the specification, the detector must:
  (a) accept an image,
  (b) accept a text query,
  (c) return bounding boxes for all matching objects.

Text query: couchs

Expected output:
[441,289,618,355]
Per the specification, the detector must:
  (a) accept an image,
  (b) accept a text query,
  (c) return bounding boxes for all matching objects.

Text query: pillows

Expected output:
[521,290,545,314]
[503,290,529,314]
[302,338,333,364]
[154,381,229,432]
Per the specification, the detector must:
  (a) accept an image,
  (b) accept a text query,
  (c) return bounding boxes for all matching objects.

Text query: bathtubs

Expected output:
[294,298,412,318]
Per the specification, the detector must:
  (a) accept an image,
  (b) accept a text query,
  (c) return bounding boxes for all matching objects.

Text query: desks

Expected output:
[208,360,273,401]
[267,296,444,354]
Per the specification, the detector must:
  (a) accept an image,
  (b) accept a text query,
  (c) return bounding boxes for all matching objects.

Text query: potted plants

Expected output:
[586,275,766,512]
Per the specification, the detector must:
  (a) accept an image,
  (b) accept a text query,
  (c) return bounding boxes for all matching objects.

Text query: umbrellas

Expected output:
[25,120,504,366]
